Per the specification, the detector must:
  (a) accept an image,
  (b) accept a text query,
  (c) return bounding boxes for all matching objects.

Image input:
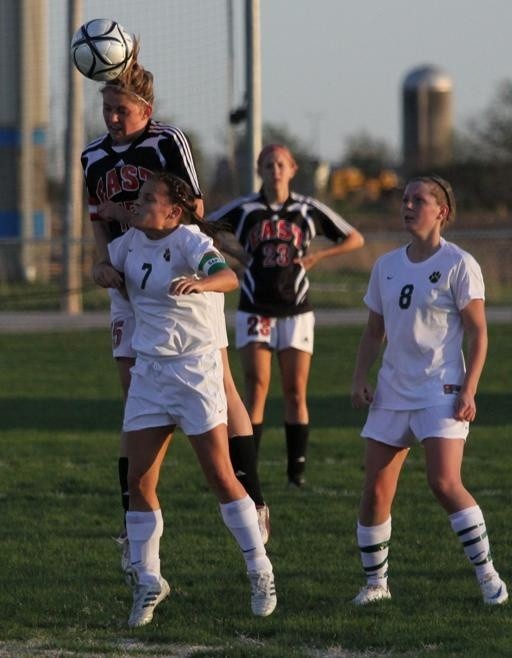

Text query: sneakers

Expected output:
[128,575,171,629]
[255,501,271,544]
[348,583,392,607]
[116,531,134,574]
[289,468,305,491]
[477,570,508,606]
[246,559,277,617]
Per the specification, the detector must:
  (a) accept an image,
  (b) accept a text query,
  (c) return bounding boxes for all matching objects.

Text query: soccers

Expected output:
[71,19,135,81]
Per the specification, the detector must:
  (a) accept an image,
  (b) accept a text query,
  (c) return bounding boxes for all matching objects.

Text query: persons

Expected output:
[201,139,364,492]
[81,61,272,551]
[348,171,509,610]
[85,167,278,629]
